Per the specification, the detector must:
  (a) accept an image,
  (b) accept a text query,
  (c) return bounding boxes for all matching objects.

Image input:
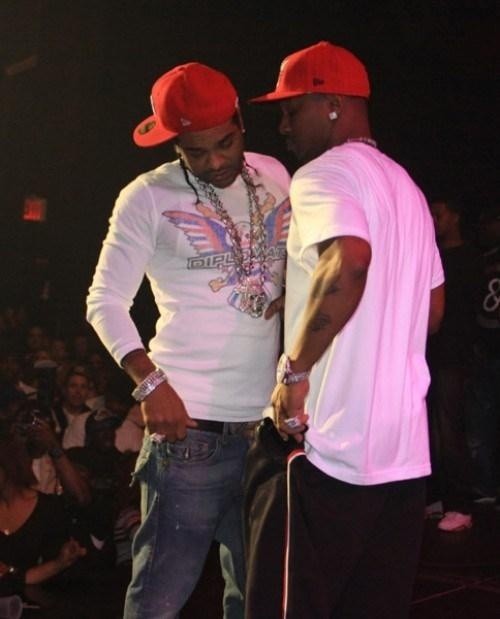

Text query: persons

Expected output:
[241,38,446,619]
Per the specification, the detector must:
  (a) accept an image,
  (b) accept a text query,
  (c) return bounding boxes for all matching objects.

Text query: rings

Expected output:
[283,415,302,430]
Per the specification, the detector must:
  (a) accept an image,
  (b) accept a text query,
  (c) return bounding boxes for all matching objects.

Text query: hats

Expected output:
[131,62,239,148]
[247,40,371,102]
[85,407,124,432]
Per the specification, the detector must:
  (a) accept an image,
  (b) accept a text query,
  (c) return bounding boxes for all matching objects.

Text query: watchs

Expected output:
[83,60,293,618]
[276,353,311,384]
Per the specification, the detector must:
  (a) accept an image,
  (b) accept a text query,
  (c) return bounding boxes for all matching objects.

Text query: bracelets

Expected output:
[130,367,169,402]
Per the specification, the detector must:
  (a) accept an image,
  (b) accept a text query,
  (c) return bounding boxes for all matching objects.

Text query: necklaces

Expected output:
[192,167,255,275]
[199,166,267,292]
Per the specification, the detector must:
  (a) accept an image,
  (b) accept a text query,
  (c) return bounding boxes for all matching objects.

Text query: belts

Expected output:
[184,416,258,437]
